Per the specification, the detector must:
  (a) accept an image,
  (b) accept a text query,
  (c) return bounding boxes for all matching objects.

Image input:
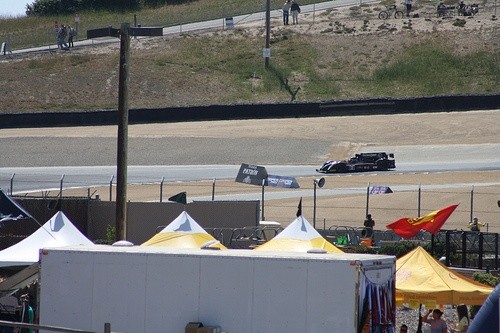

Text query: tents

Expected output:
[251,214,346,254]
[0,211,95,268]
[139,210,229,249]
[394,245,495,333]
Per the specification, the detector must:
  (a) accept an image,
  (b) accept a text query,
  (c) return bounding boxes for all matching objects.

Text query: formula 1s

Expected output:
[316,153,396,173]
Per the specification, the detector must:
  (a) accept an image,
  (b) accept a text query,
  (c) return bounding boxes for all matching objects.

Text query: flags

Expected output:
[386,204,459,238]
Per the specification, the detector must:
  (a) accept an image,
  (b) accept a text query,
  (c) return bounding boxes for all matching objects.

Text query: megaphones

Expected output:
[313,178,326,187]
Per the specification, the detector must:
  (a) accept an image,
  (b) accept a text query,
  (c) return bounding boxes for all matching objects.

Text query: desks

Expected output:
[336,243,381,253]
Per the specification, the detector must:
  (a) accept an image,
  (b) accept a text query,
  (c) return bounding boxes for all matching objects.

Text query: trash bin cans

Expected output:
[225,16,233,27]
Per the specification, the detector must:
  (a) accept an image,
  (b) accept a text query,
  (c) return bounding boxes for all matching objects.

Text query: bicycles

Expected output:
[378,5,404,20]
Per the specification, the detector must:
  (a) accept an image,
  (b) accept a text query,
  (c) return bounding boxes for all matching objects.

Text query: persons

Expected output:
[57,24,75,49]
[290,0,301,25]
[437,2,447,17]
[400,324,408,333]
[456,1,466,16]
[422,309,448,333]
[403,0,412,17]
[282,0,291,25]
[467,217,486,241]
[363,213,375,230]
[450,304,469,333]
